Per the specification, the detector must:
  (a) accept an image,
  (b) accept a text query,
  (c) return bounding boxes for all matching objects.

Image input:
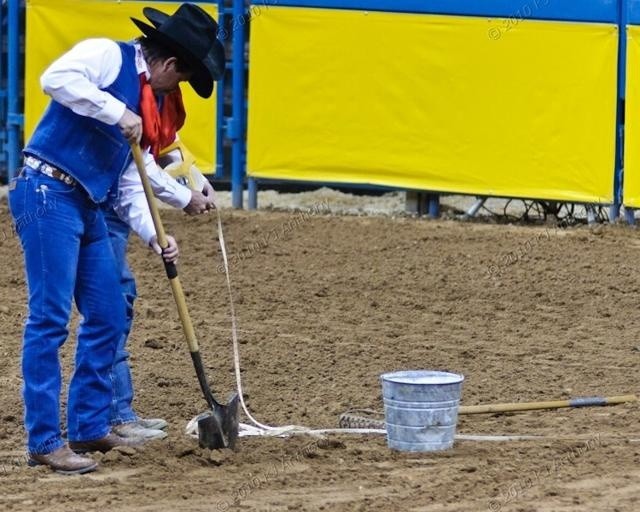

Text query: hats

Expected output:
[130,3,226,99]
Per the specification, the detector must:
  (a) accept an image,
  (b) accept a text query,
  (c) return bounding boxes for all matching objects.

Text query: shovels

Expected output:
[132,141,239,451]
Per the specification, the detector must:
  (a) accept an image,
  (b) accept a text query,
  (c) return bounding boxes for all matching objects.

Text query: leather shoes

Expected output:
[27,419,168,474]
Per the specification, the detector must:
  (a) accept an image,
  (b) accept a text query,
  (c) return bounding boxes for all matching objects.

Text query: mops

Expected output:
[338,394,638,430]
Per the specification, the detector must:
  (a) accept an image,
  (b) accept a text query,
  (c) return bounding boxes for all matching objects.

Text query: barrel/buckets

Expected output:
[379,370,464,452]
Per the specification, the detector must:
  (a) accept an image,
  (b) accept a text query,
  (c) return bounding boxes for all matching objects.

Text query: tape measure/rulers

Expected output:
[161,161,537,441]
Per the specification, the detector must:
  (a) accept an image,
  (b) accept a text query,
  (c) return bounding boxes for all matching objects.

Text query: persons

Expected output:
[4,10,215,474]
[75,2,230,443]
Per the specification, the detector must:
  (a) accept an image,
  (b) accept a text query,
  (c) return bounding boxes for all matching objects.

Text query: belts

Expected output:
[25,156,76,187]
